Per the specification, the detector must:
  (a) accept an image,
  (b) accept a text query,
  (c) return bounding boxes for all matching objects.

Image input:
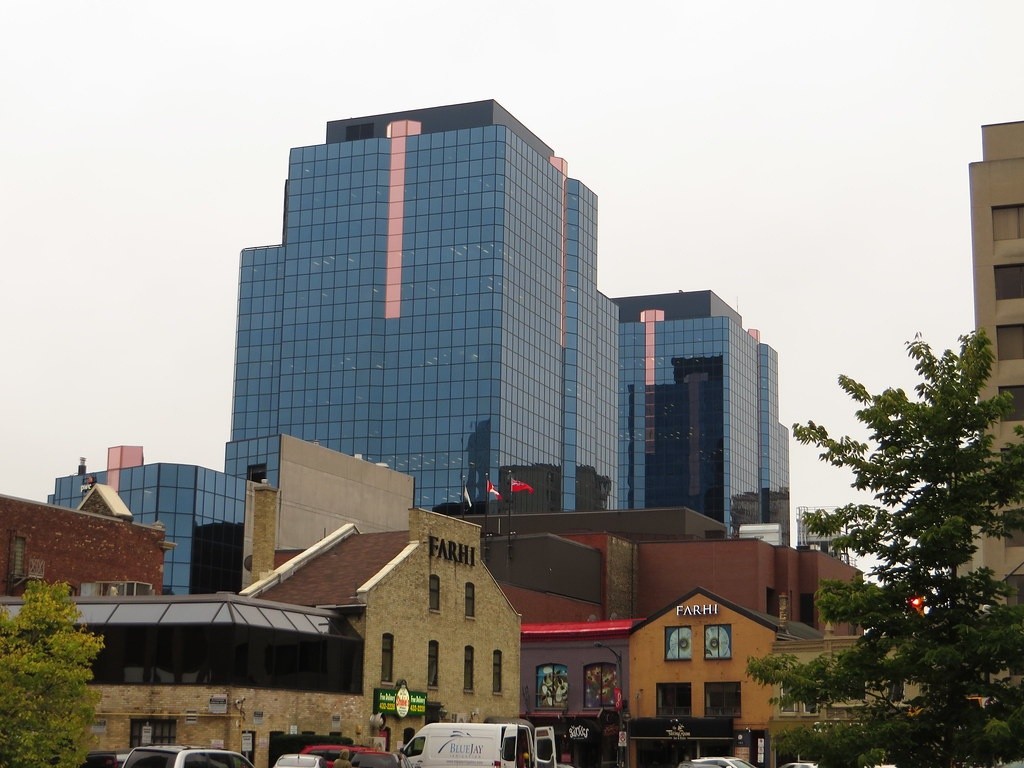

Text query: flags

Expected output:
[510,475,534,495]
[486,480,502,501]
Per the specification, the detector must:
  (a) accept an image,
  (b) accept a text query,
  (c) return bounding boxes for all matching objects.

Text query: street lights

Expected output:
[594,642,623,768]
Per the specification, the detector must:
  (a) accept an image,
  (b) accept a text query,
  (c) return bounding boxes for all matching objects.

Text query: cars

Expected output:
[677,761,722,768]
[117,754,129,768]
[273,754,326,768]
[779,763,818,768]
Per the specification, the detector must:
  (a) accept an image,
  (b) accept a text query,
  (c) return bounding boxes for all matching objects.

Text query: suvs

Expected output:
[351,751,413,768]
[80,751,118,768]
[691,757,758,768]
[121,745,255,768]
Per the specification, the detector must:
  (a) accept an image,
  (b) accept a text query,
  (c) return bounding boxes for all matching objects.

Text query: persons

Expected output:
[333,749,352,768]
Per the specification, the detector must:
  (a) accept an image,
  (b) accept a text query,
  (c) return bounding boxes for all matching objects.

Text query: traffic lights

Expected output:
[908,594,925,618]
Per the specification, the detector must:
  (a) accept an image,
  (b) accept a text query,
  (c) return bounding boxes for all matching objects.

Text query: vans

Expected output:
[300,746,377,768]
[400,722,557,768]
